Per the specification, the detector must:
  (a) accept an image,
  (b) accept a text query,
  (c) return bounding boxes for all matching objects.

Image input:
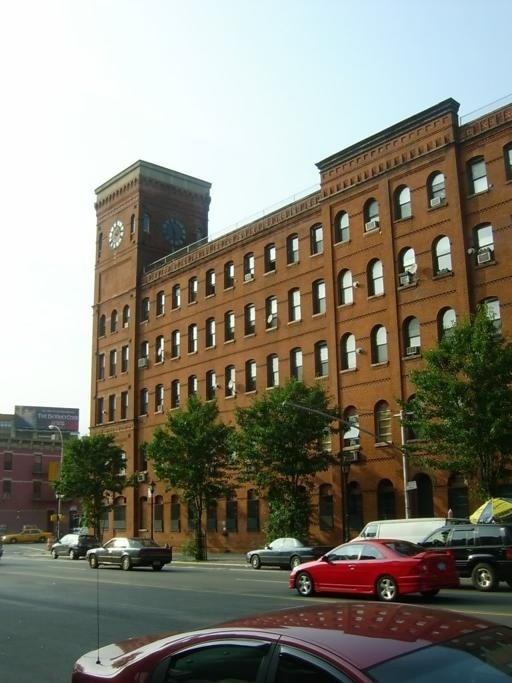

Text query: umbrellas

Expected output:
[469,494,512,526]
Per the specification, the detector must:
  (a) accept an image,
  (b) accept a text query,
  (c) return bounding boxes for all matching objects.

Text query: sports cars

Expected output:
[289,538,459,601]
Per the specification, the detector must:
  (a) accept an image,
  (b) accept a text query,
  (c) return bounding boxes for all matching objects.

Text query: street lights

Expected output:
[48,424,64,540]
[148,477,156,540]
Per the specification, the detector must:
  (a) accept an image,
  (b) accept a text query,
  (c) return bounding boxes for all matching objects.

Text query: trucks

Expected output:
[347,516,472,548]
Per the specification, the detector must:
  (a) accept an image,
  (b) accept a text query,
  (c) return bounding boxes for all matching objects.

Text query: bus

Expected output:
[71,513,88,535]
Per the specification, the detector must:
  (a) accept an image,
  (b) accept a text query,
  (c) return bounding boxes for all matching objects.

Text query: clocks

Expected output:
[106,219,125,248]
[162,216,188,246]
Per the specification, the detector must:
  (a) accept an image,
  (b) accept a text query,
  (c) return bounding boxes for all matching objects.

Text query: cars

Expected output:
[246,535,335,570]
[86,535,173,572]
[0,524,55,544]
[49,532,100,561]
[73,605,512,682]
[0,539,4,557]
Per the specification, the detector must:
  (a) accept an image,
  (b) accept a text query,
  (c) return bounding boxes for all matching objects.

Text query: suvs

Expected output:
[416,522,511,591]
